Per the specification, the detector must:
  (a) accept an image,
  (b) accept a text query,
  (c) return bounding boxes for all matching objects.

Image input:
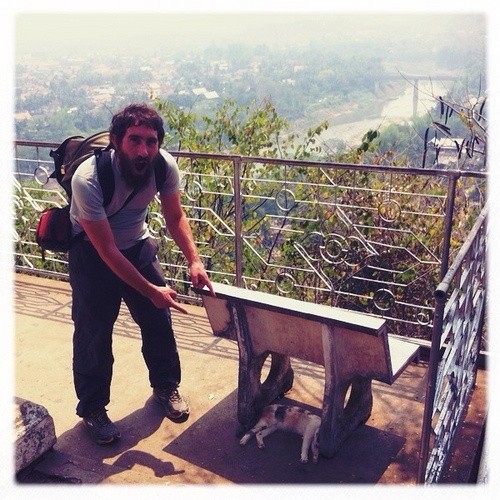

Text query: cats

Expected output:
[239,403,320,464]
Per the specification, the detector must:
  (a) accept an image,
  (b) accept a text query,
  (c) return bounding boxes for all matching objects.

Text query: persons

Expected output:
[68,103,216,444]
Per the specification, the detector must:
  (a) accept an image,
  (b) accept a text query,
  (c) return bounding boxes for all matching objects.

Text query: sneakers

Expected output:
[153,383,189,421]
[83,407,121,444]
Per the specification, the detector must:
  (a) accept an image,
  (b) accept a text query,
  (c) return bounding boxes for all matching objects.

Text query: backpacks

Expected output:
[35,131,168,253]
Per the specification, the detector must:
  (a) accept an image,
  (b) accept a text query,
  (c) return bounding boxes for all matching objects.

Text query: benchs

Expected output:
[190,281,421,459]
